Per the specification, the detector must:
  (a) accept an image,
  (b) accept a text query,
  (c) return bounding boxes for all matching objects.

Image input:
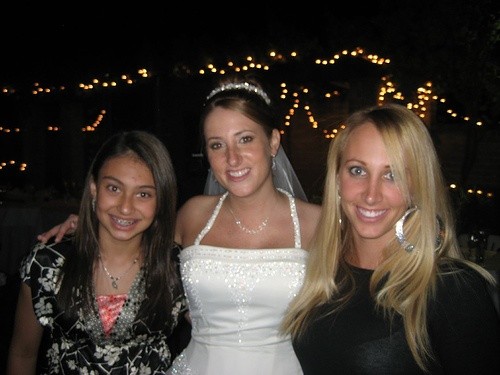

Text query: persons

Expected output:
[278,104,500,375]
[38,82,325,375]
[7,130,192,375]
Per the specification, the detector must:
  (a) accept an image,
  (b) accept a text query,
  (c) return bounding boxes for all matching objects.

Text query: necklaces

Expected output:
[226,197,280,234]
[99,251,139,289]
